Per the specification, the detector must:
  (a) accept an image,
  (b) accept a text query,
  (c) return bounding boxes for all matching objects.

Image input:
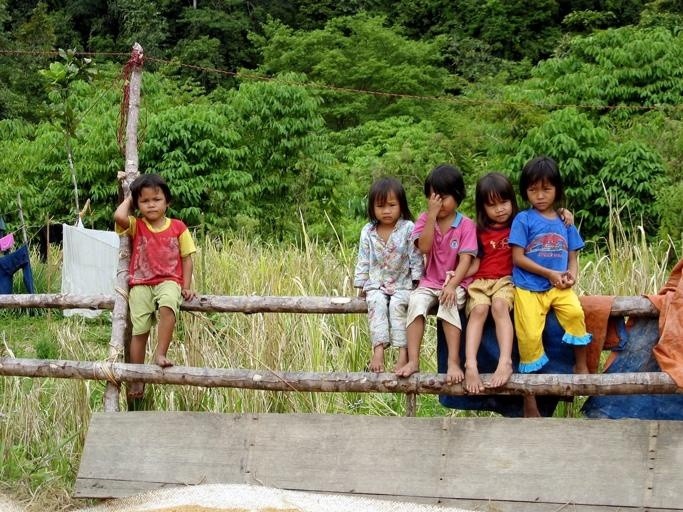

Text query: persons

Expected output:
[113,174,198,397]
[355,176,425,373]
[509,157,593,418]
[394,164,478,385]
[442,173,574,394]
